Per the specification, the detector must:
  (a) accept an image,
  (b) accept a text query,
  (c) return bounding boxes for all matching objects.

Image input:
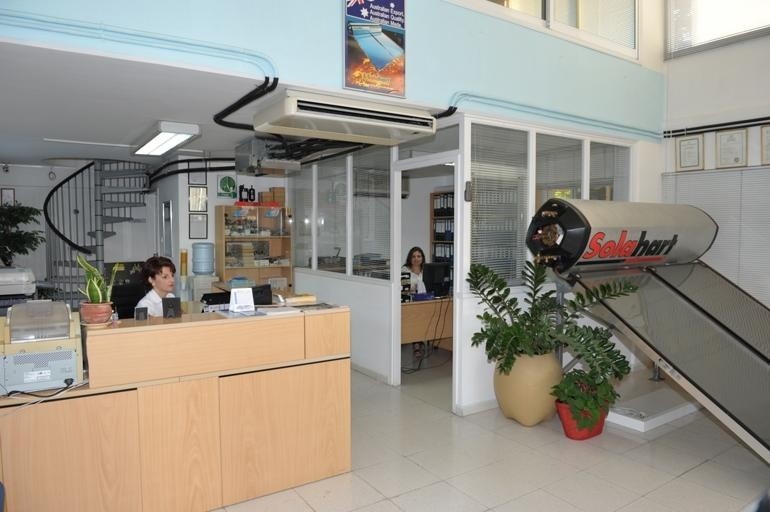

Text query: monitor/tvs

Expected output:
[423,262,450,297]
[202,284,272,313]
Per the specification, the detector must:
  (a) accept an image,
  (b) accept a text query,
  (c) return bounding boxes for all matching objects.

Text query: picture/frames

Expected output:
[675,132,705,173]
[189,185,208,212]
[189,213,208,239]
[715,127,748,169]
[760,125,770,166]
[188,172,207,185]
[162,199,174,259]
[0,188,16,207]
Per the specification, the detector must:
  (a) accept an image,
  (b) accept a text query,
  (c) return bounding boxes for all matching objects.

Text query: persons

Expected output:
[401,247,427,294]
[135,256,176,317]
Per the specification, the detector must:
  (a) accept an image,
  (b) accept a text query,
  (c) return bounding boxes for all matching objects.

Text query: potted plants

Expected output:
[75,253,125,322]
[548,367,620,440]
[465,256,637,426]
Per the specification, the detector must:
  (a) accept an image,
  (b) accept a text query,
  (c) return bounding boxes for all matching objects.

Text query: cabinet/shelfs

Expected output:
[215,204,294,296]
[431,189,517,270]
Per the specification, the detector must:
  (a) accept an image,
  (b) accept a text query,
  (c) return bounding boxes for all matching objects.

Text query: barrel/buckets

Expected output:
[192,242,214,275]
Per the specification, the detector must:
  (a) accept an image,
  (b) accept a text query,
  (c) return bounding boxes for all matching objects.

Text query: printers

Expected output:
[0,299,84,395]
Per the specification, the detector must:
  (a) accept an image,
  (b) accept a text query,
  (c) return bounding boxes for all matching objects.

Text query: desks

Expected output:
[401,295,453,345]
[320,265,389,278]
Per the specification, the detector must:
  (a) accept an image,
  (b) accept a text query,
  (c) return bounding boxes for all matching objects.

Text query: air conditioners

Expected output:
[352,169,409,200]
[251,88,436,145]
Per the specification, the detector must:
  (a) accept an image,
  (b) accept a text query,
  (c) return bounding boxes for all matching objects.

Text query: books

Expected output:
[257,306,303,315]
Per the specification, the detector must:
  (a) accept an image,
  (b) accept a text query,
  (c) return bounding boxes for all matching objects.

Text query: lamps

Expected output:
[130,121,202,159]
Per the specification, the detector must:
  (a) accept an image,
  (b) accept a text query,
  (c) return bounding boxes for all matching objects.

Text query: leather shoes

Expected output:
[414,349,422,360]
